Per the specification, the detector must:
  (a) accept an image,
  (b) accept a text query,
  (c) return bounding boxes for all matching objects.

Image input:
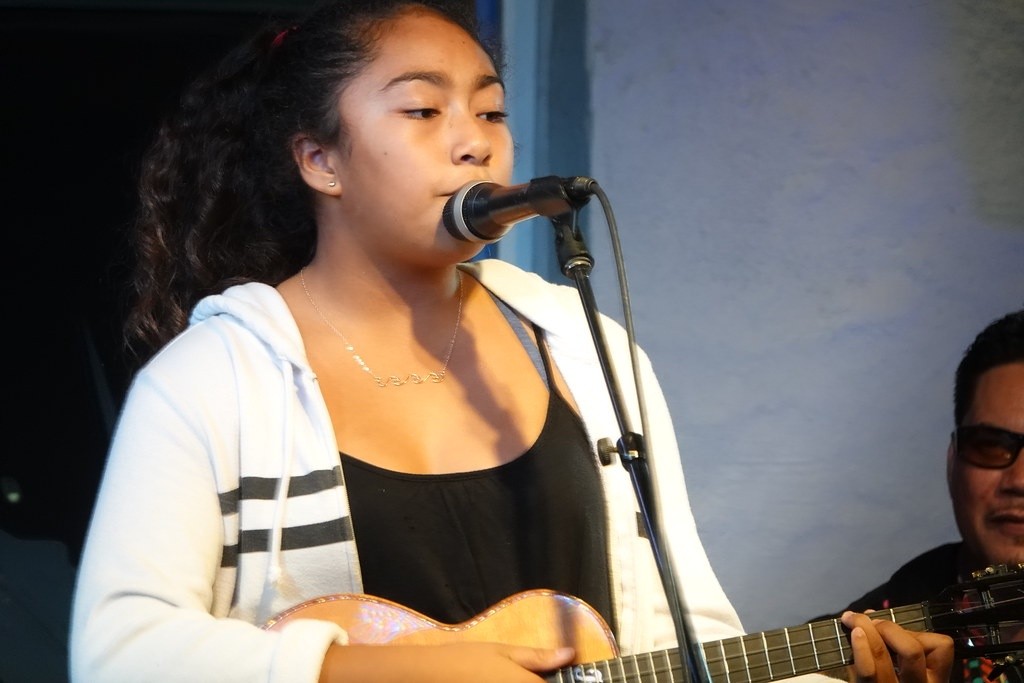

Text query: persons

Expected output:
[804,309,1024,683]
[63,0,959,683]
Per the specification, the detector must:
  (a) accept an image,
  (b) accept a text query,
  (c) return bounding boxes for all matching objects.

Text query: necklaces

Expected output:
[296,263,464,388]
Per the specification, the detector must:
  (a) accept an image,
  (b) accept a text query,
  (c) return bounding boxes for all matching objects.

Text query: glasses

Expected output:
[950,422,1024,469]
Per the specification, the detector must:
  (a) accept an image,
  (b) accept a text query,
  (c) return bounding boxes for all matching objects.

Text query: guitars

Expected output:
[262,559,1024,683]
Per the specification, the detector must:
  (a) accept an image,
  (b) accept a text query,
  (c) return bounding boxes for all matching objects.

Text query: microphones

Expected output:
[442,175,598,248]
